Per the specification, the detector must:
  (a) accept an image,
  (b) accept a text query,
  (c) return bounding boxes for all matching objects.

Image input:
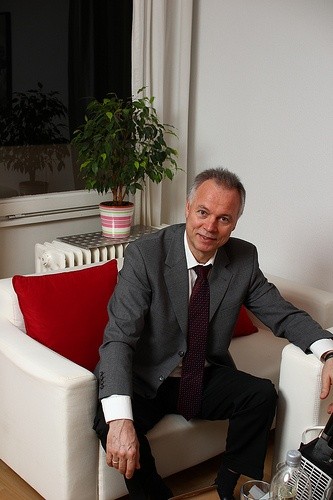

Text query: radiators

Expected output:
[33,223,171,274]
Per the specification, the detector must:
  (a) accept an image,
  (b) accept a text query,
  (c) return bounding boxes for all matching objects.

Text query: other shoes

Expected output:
[215,468,241,500]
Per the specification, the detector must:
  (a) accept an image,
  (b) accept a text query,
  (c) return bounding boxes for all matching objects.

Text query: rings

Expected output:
[112,460,119,464]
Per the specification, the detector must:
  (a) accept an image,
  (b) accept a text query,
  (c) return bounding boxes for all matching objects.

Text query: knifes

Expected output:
[312,412,333,466]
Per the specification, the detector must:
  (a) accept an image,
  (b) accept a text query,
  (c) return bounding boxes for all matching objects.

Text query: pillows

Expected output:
[12,259,118,374]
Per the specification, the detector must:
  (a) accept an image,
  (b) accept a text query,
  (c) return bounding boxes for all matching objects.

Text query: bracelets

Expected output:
[325,352,333,362]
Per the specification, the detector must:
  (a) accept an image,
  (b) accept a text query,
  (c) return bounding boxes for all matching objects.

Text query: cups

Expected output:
[239,480,271,500]
[275,461,286,472]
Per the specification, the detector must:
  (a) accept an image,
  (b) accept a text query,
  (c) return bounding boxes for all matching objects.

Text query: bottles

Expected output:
[269,450,312,500]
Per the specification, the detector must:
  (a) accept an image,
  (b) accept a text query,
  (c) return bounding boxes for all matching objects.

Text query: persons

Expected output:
[94,166,333,500]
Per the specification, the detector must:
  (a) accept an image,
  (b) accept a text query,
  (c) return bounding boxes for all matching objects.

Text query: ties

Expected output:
[178,264,212,422]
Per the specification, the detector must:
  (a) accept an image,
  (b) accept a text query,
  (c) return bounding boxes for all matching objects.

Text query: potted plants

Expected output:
[71,86,183,239]
[0,81,74,196]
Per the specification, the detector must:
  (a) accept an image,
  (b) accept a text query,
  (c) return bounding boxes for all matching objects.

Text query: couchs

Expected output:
[0,257,333,500]
